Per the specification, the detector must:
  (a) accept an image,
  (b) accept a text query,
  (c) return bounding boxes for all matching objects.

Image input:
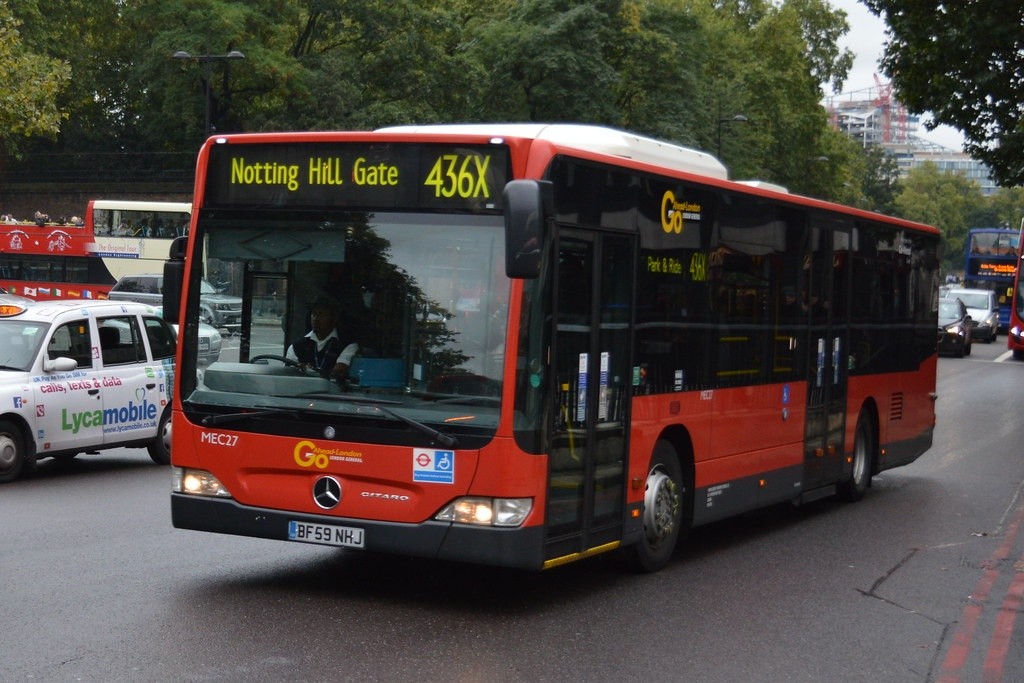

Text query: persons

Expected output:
[282,295,360,382]
[5,210,84,227]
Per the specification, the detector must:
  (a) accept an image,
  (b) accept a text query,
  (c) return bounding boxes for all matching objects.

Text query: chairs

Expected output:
[138,327,168,359]
[94,327,122,364]
[99,225,188,239]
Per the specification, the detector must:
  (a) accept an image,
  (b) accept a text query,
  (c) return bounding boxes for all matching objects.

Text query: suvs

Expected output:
[109,274,252,336]
[946,288,1000,344]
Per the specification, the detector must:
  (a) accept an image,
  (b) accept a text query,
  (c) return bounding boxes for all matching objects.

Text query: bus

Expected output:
[1007,216,1024,361]
[1,200,209,302]
[170,125,941,575]
[963,228,1024,335]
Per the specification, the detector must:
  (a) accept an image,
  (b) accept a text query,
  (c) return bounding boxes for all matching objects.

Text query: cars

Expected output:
[153,306,223,365]
[939,297,972,358]
[0,301,206,484]
